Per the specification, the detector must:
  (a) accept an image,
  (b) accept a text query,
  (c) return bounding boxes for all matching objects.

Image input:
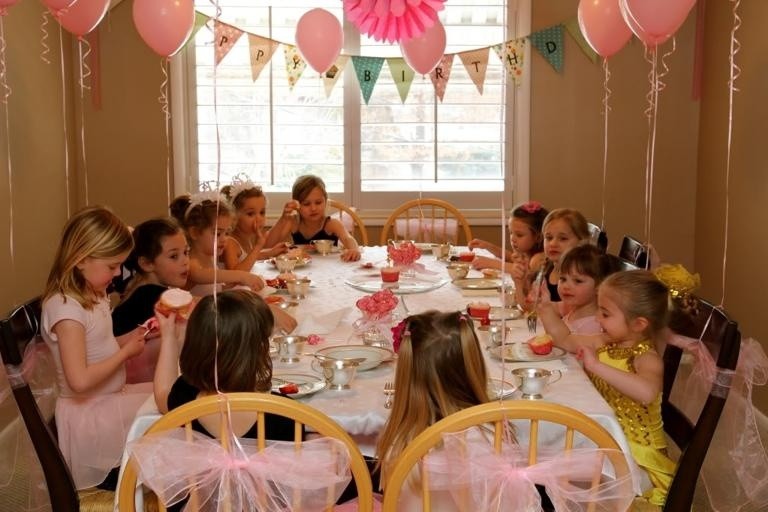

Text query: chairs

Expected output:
[660,294,742,511]
[381,198,474,245]
[382,399,634,511]
[323,199,368,247]
[3,294,119,510]
[584,220,653,273]
[117,390,373,510]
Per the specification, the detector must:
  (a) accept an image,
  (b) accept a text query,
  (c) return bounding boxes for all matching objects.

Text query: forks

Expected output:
[382,381,394,407]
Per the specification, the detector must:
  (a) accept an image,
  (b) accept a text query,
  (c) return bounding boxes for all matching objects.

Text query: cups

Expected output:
[309,238,334,256]
[509,367,563,399]
[311,355,365,390]
[387,239,414,259]
[380,268,400,283]
[266,254,312,316]
[430,243,449,259]
[444,263,469,282]
[461,252,476,263]
[268,335,308,365]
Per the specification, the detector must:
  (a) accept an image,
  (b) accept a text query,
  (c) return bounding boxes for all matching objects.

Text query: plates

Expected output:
[346,270,450,297]
[314,345,396,372]
[271,372,325,400]
[412,242,441,252]
[484,378,514,400]
[451,276,567,362]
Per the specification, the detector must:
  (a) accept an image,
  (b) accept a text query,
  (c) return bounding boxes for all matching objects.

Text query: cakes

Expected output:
[381,266,400,282]
[161,288,191,311]
[462,251,475,262]
[467,302,491,318]
[529,334,552,355]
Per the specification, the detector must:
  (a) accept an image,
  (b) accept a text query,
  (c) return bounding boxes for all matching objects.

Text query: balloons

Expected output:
[42,0,77,12]
[131,0,195,58]
[577,0,631,59]
[624,0,696,38]
[398,17,447,75]
[295,8,342,73]
[51,0,112,36]
[619,0,687,48]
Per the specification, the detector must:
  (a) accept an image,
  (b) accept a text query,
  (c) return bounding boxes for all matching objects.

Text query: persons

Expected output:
[528,264,701,512]
[216,171,290,272]
[368,311,532,511]
[169,180,264,292]
[264,174,361,262]
[40,207,154,489]
[154,287,305,511]
[113,215,298,337]
[469,203,627,372]
